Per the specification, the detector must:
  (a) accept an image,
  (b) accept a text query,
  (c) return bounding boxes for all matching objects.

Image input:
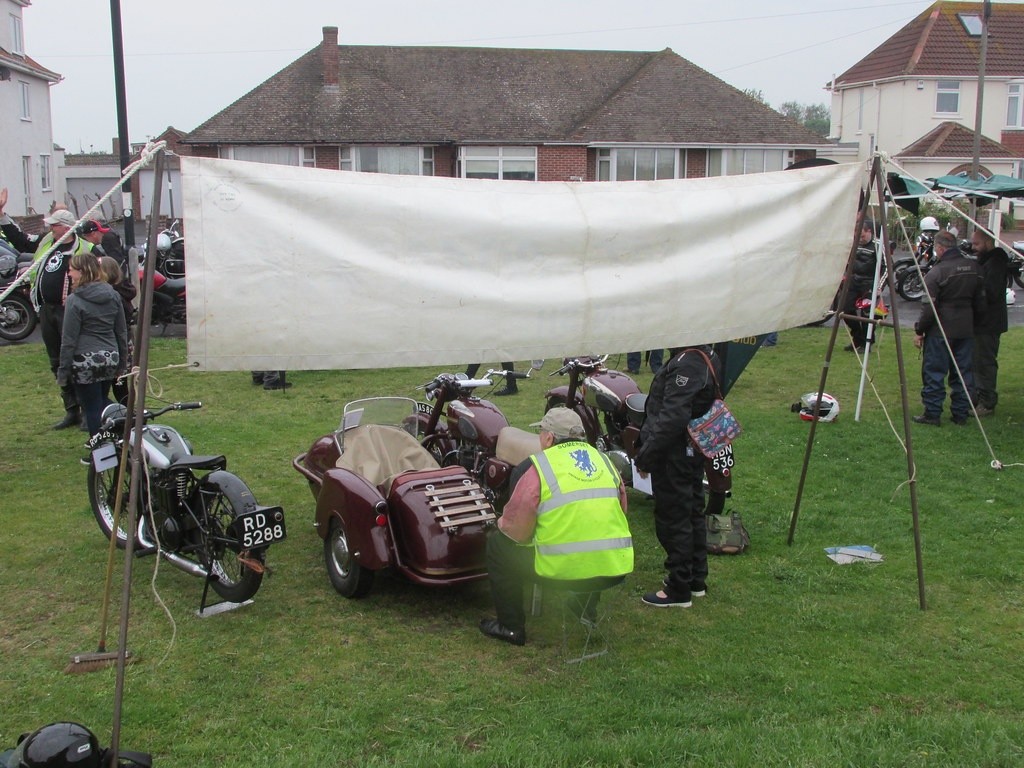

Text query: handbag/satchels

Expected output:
[686,399,742,460]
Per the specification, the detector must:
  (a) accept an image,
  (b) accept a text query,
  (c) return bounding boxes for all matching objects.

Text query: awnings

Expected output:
[855,167,1024,425]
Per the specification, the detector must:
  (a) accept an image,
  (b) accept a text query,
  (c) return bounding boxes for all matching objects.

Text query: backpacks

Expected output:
[705,511,751,555]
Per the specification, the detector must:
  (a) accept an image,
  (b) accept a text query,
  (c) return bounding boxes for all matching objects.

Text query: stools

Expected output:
[526,586,613,669]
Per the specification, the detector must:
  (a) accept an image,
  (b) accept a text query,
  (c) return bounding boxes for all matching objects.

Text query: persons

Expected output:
[911,231,987,426]
[251,370,292,390]
[626,350,664,375]
[463,363,520,397]
[844,221,895,353]
[634,345,722,609]
[1,185,138,466]
[968,229,1008,417]
[479,408,634,646]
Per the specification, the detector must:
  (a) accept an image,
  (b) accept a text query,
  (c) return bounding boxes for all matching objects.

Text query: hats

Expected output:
[528,408,586,440]
[42,210,76,226]
[82,220,111,233]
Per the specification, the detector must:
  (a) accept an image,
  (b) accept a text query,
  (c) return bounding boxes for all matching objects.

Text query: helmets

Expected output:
[800,391,839,423]
[156,233,171,251]
[0,254,18,278]
[875,307,888,319]
[920,216,940,231]
[1006,288,1015,305]
[855,292,871,308]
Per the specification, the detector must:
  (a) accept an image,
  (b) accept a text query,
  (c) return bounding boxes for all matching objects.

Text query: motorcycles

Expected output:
[0,219,188,342]
[292,356,542,600]
[544,354,737,516]
[886,216,1024,302]
[86,401,287,619]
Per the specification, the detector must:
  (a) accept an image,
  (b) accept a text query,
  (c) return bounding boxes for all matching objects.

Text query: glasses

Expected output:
[49,223,62,227]
[535,427,543,434]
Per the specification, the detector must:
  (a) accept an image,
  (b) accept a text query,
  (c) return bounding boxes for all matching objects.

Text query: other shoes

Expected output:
[263,378,292,390]
[52,414,82,429]
[968,403,994,417]
[253,379,264,385]
[632,369,639,374]
[494,384,518,395]
[85,441,91,449]
[479,618,526,647]
[845,342,858,351]
[857,346,871,353]
[652,369,657,373]
[80,457,90,466]
[80,415,88,431]
[912,411,941,427]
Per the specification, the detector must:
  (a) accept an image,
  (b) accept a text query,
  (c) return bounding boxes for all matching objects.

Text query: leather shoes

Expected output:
[663,577,706,597]
[641,588,691,608]
